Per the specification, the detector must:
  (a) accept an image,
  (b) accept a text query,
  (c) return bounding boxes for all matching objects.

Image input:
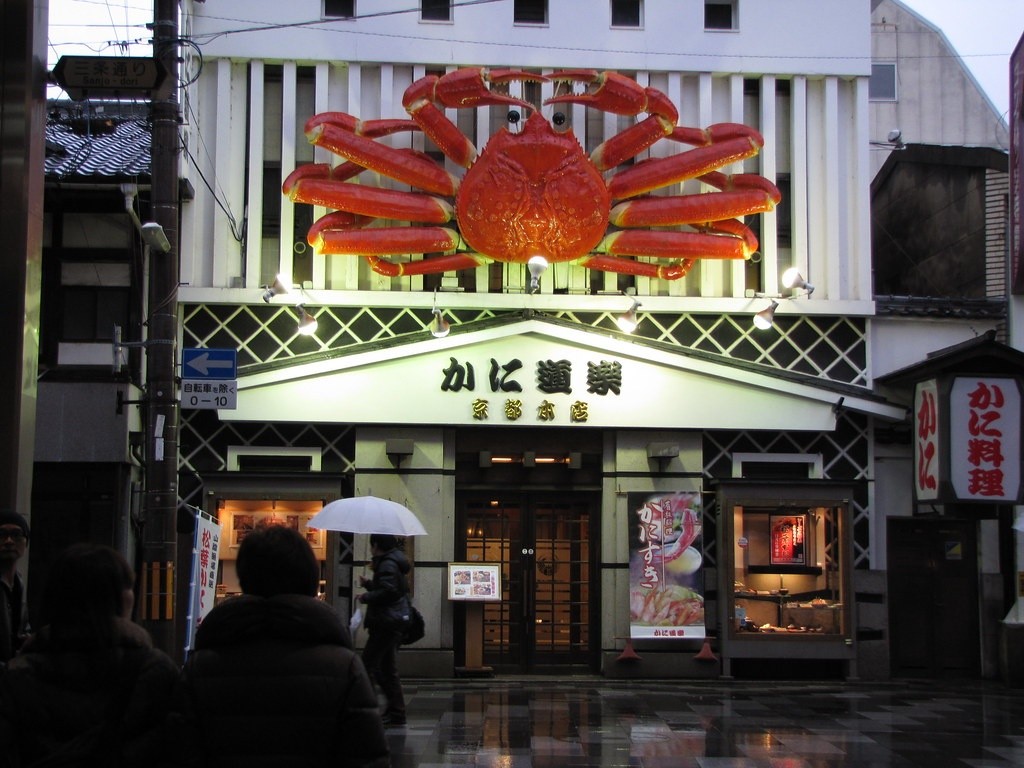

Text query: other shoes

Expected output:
[381,716,408,728]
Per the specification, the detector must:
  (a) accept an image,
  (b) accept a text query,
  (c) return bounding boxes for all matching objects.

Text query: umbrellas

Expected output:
[305,496,427,589]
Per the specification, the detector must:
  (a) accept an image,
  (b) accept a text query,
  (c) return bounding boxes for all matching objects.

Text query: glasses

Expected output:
[0,529,27,538]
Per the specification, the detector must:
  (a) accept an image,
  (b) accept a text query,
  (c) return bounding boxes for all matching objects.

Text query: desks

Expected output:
[734,592,839,629]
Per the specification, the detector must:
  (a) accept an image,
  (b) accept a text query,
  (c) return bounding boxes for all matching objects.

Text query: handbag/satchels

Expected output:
[392,605,425,645]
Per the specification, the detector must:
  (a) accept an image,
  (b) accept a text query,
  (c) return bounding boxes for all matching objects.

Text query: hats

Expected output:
[0,510,29,538]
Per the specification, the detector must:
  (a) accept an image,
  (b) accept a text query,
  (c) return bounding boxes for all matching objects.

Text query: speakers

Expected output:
[646,441,679,458]
[386,439,414,455]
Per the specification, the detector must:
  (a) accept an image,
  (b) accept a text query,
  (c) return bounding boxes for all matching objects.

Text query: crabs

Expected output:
[283,68,782,282]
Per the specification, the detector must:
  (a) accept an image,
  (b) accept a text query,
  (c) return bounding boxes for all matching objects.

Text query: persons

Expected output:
[0,511,30,670]
[185,527,396,768]
[360,534,411,726]
[0,547,205,768]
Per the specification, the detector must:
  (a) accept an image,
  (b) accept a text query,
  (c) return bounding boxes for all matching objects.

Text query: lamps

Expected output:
[782,268,815,300]
[618,291,642,333]
[753,295,779,331]
[869,130,907,151]
[431,286,450,338]
[528,256,548,294]
[297,301,319,337]
[261,275,289,303]
